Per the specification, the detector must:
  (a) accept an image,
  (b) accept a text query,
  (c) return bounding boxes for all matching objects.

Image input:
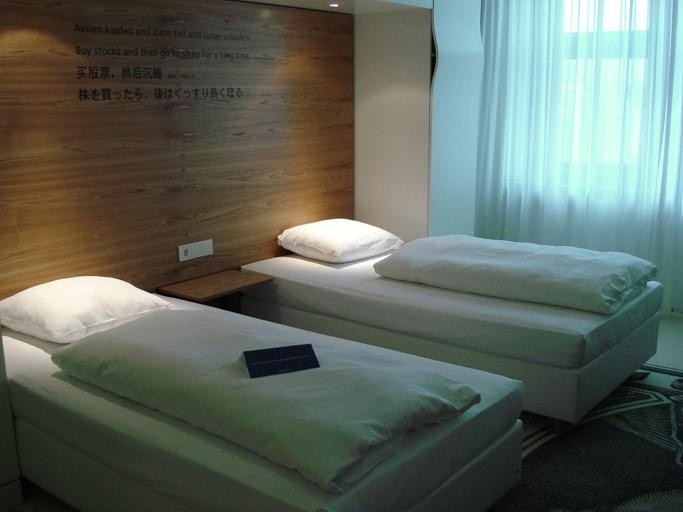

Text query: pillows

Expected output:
[274,216,403,261]
[2,274,177,347]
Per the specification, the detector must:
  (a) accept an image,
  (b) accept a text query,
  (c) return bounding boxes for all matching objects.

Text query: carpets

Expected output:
[491,360,683,510]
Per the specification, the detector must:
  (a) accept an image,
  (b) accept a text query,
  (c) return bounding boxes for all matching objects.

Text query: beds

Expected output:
[239,240,664,429]
[3,290,532,512]
